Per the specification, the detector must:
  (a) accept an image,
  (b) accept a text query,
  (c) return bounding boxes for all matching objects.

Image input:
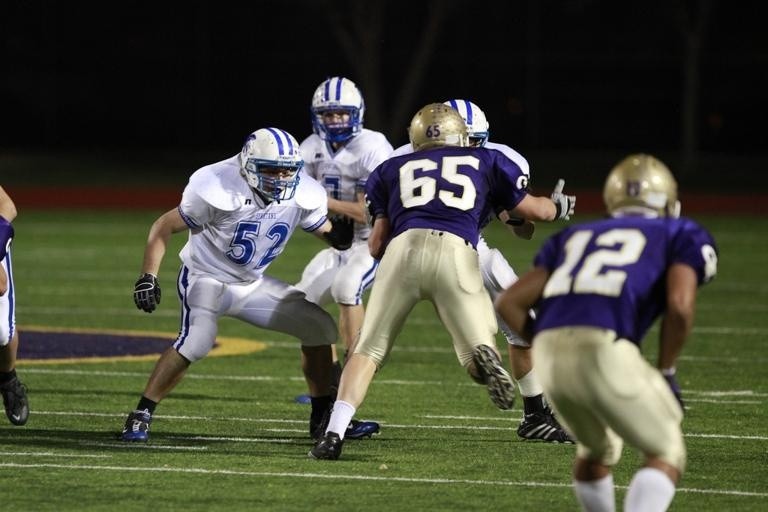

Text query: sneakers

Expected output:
[470,343,516,411]
[516,396,576,445]
[307,414,380,459]
[122,409,152,442]
[0,376,28,425]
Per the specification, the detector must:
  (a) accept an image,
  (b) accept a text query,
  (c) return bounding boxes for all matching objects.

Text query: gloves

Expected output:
[322,212,355,251]
[550,178,576,223]
[134,270,162,313]
[660,365,687,415]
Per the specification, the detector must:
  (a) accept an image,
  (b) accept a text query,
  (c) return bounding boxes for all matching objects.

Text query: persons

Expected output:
[343,95,575,445]
[118,125,378,442]
[0,183,30,427]
[489,153,718,512]
[287,74,396,406]
[305,102,574,462]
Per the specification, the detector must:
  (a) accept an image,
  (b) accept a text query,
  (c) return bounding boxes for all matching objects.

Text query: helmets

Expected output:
[239,127,306,201]
[603,153,682,219]
[311,77,365,143]
[408,98,490,153]
